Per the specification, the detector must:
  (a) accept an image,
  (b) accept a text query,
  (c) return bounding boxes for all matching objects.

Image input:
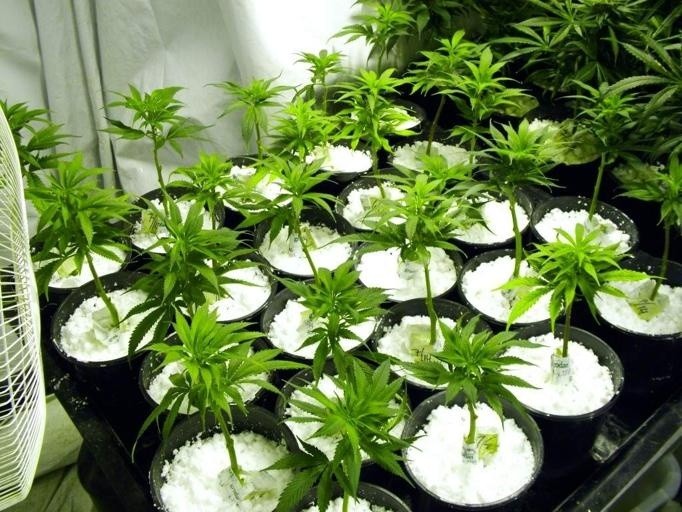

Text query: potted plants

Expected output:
[1,1,681,511]
